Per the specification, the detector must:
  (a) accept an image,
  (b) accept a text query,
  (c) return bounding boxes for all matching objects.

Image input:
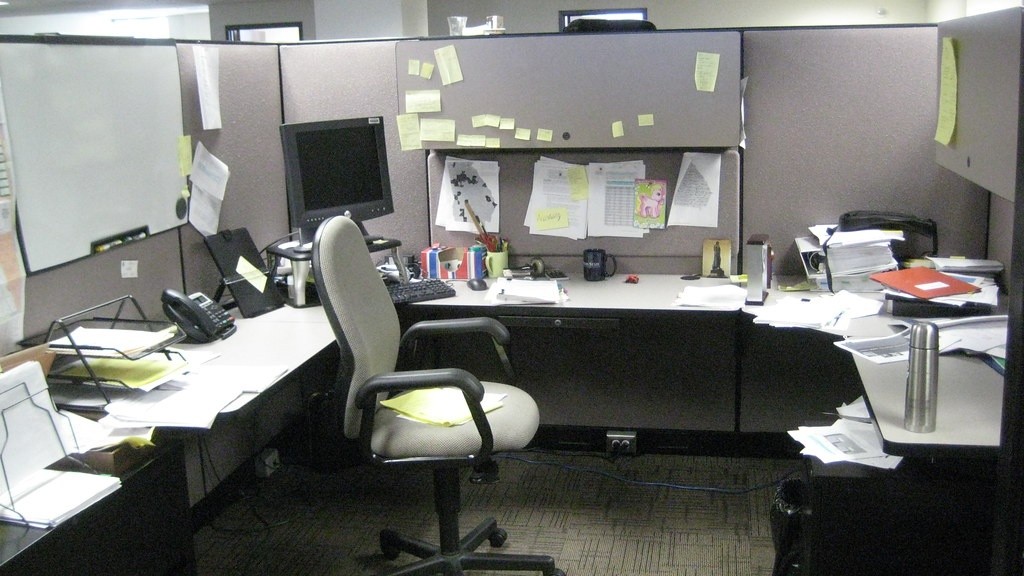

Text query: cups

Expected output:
[484,251,509,278]
[582,248,617,281]
[486,16,503,29]
[446,16,468,35]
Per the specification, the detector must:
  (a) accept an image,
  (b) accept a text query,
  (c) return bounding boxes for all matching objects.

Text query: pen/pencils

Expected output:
[497,237,509,252]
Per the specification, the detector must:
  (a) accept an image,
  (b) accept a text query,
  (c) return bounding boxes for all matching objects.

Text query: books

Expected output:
[885,288,991,318]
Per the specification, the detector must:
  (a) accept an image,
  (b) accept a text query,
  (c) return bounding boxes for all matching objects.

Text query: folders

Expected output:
[869,265,982,302]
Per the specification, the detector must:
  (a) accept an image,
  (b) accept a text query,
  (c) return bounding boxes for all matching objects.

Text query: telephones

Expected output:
[161,286,238,342]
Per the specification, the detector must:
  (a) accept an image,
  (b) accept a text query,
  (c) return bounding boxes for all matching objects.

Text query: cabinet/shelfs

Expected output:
[801,458,996,576]
[0,441,200,576]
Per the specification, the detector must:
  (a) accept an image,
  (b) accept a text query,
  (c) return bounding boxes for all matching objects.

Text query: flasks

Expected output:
[904,321,939,433]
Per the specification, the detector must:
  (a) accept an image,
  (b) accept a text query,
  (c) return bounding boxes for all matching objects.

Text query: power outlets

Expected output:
[606,432,637,456]
[256,450,281,476]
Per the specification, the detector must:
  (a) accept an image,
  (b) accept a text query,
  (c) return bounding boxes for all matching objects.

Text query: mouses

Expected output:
[467,279,487,290]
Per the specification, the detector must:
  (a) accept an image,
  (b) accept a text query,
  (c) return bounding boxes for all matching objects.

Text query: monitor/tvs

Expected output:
[278,117,395,253]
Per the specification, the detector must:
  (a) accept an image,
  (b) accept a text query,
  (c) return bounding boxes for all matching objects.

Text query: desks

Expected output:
[0,277,1005,576]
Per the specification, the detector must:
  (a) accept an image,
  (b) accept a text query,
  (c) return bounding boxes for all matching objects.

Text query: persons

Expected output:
[714,242,720,269]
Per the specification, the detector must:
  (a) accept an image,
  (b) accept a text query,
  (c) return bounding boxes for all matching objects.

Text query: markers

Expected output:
[122,232,147,243]
[94,239,123,251]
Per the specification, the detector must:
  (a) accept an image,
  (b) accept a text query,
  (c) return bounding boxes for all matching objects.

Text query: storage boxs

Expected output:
[75,410,153,478]
[421,243,488,281]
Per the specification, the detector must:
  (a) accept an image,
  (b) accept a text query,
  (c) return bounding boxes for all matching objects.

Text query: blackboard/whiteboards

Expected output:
[0,35,193,278]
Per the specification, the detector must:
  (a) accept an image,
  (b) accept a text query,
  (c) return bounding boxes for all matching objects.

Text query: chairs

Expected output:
[312,216,565,576]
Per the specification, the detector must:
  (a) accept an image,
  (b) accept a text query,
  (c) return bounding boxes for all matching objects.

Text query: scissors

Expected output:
[479,234,496,252]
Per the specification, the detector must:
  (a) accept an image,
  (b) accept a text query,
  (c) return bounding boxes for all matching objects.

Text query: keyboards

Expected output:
[386,279,456,304]
